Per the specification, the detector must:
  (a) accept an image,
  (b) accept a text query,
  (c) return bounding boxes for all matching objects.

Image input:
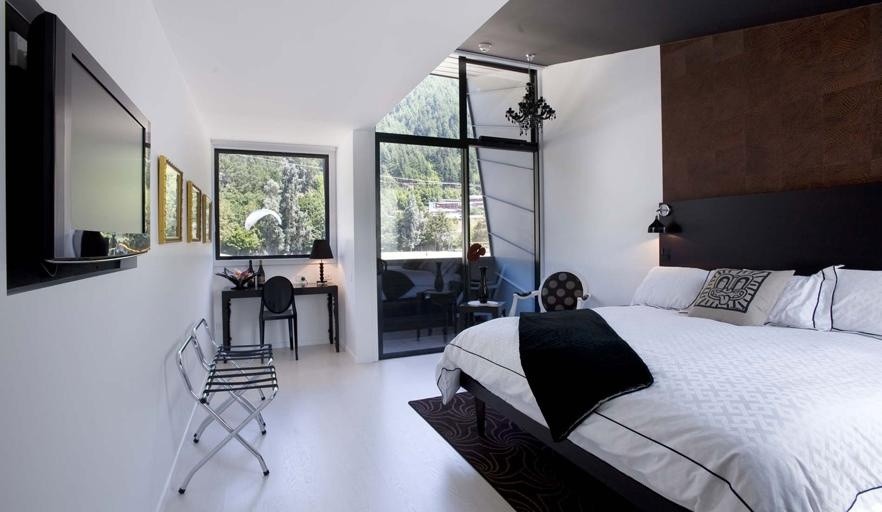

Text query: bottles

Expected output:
[259,260,266,288]
[247,259,256,287]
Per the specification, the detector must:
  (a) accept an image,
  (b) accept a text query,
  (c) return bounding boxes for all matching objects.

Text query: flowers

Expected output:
[466,243,486,266]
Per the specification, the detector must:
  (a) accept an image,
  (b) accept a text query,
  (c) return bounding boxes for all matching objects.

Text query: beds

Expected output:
[431,184,882,511]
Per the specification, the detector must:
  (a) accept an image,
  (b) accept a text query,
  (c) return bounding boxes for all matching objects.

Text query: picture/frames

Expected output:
[187,180,202,243]
[202,193,214,244]
[157,154,184,245]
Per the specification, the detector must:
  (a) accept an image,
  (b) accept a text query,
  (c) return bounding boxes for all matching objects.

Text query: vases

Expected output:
[477,264,489,304]
[434,260,445,291]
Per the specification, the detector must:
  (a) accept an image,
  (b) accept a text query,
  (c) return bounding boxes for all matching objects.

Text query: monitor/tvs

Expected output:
[24,10,152,266]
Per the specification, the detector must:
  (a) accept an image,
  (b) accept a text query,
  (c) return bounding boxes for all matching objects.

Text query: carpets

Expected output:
[408,391,699,512]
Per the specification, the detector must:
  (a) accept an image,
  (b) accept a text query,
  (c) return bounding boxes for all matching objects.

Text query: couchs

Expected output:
[378,255,505,333]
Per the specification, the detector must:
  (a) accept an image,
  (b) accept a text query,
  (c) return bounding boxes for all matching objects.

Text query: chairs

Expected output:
[258,275,299,364]
[174,335,279,494]
[509,269,591,316]
[190,317,274,436]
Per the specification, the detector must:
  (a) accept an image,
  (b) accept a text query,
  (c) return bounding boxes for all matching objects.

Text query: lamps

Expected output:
[504,53,556,138]
[308,239,336,284]
[645,202,685,236]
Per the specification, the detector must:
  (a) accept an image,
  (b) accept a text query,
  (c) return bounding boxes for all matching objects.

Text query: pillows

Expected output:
[678,267,796,327]
[764,264,846,332]
[381,269,416,302]
[831,268,882,340]
[629,263,710,313]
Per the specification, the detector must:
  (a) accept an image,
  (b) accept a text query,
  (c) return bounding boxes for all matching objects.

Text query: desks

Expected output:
[416,289,458,342]
[221,280,342,354]
[460,298,507,330]
